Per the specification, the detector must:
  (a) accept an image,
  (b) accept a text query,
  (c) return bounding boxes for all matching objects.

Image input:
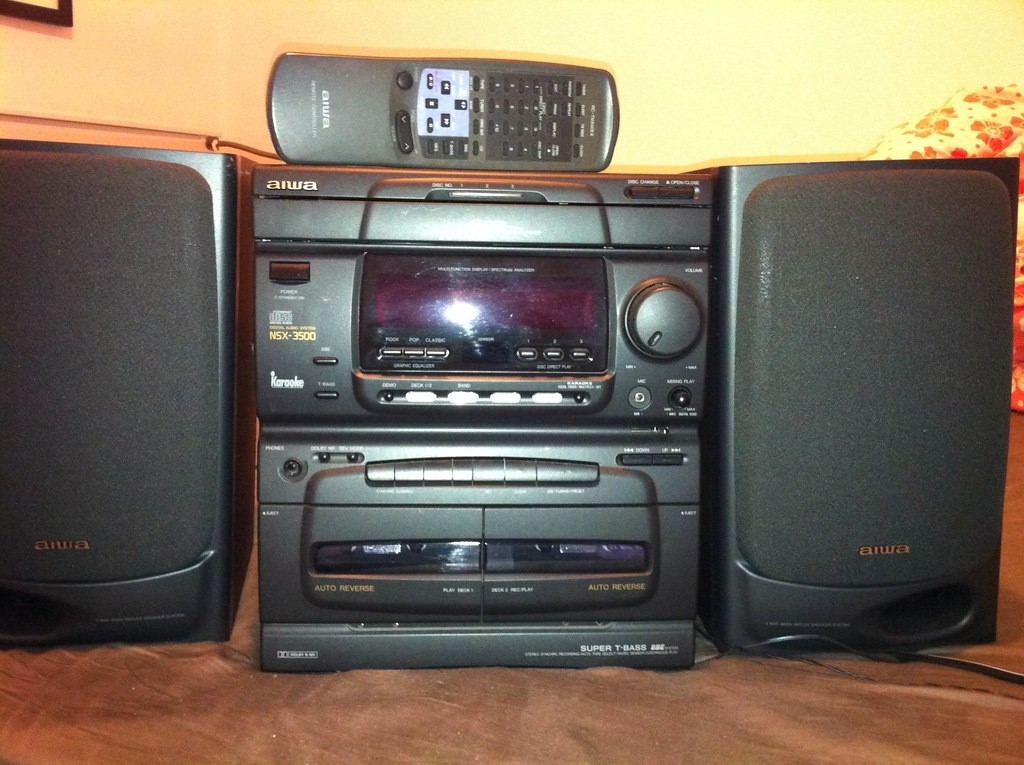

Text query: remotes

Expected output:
[266,50,620,173]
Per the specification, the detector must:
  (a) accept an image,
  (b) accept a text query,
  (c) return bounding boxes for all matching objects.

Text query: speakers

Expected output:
[678,157,1019,660]
[0,140,259,654]
